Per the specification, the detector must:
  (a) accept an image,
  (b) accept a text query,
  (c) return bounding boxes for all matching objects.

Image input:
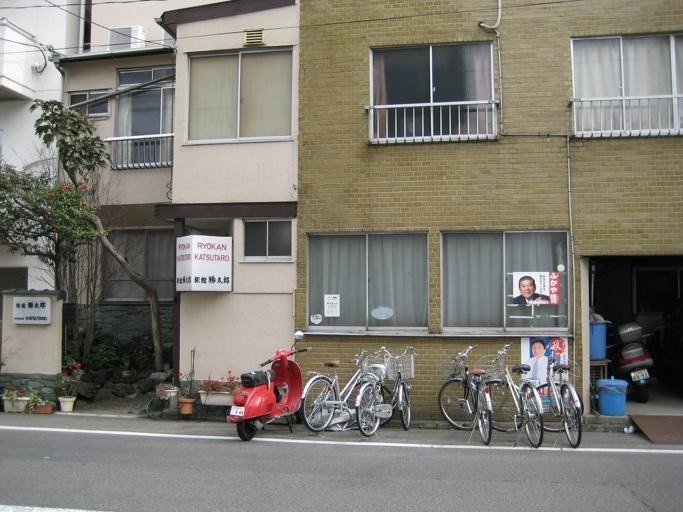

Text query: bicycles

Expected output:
[476,340,545,448]
[354,343,418,438]
[301,340,395,434]
[526,342,584,449]
[436,342,493,446]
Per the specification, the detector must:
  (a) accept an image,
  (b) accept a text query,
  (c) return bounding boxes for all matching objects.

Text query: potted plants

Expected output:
[178,369,198,415]
[54,372,83,413]
[62,353,72,376]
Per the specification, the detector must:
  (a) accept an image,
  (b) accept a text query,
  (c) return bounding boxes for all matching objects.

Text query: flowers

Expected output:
[0,384,49,416]
[155,381,176,398]
[199,370,241,394]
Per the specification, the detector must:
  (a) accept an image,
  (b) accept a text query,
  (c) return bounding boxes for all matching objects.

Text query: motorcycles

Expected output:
[609,313,654,403]
[224,330,310,442]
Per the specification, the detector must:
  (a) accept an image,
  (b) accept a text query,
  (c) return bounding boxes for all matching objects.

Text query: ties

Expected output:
[533,362,539,379]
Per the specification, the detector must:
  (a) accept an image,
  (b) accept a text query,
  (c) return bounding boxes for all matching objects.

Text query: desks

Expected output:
[590,359,612,400]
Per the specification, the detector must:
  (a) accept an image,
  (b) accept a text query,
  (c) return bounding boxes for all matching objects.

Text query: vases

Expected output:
[165,390,177,398]
[198,391,234,406]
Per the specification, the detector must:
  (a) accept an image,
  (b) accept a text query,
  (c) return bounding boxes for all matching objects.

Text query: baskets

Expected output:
[473,355,507,379]
[388,356,415,381]
[361,355,388,375]
[558,359,581,385]
[438,360,459,379]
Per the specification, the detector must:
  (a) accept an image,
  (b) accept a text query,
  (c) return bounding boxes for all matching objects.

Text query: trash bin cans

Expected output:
[596,376,629,416]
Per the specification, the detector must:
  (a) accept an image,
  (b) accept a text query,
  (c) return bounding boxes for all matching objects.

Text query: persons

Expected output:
[526,338,552,386]
[512,275,551,306]
